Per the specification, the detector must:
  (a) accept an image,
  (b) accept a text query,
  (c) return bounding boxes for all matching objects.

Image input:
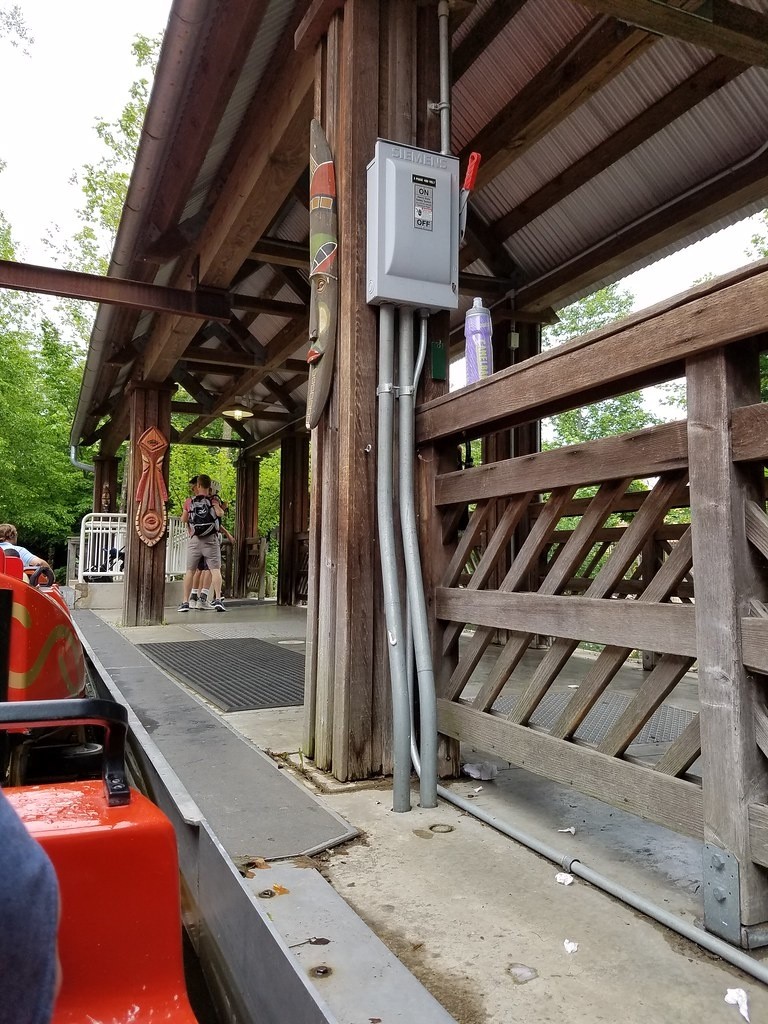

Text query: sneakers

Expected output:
[214,601,226,612]
[189,600,197,608]
[196,598,215,610]
[177,602,189,612]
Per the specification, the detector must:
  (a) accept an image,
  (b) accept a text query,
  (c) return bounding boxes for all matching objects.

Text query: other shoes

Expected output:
[210,593,225,605]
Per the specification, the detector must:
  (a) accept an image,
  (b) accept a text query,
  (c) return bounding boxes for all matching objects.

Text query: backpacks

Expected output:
[190,496,221,538]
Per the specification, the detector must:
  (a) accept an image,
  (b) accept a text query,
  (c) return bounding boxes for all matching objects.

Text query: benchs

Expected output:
[1,780,199,1024]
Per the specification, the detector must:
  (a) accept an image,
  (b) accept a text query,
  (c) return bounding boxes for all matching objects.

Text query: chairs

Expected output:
[0,544,24,580]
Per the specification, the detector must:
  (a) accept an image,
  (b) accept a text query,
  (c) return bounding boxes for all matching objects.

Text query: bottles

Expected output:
[464,296,494,386]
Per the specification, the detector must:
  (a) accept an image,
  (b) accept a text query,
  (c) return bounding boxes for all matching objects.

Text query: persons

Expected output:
[177,475,236,612]
[0,792,61,1024]
[0,524,55,583]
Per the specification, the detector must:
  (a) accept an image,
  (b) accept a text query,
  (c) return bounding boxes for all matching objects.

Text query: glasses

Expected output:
[191,485,196,492]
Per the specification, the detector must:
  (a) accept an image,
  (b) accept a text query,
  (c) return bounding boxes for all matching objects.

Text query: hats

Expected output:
[210,480,220,491]
